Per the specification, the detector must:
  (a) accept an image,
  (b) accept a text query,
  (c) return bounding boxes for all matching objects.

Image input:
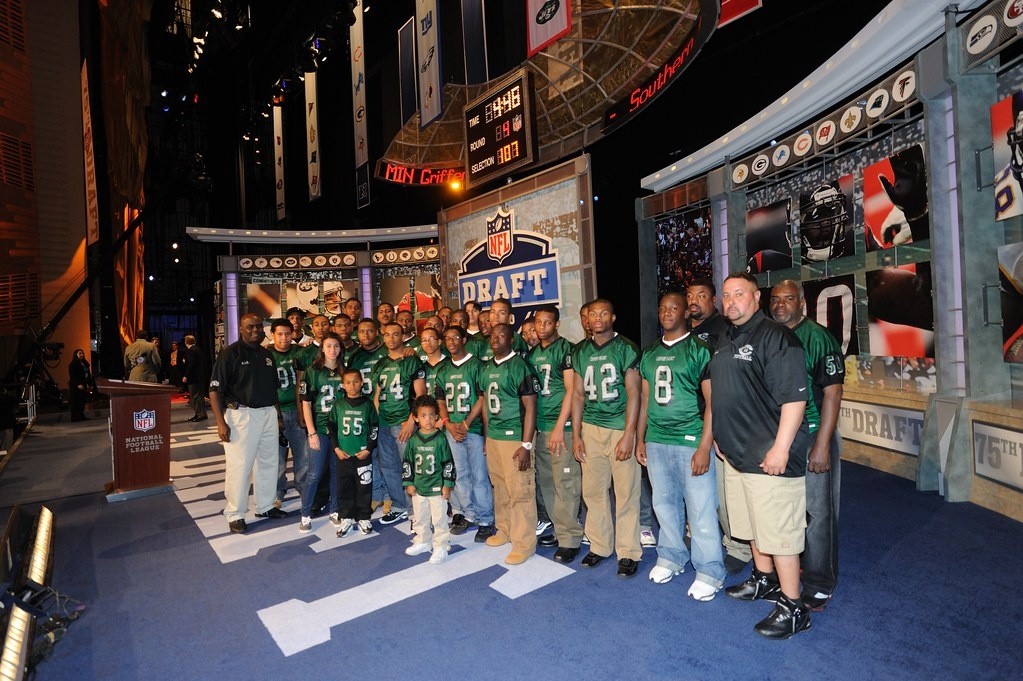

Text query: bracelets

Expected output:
[462,420,470,431]
[308,431,318,439]
[278,415,283,420]
[442,418,450,425]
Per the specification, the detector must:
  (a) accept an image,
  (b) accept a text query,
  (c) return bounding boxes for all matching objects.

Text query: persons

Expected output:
[209,312,289,534]
[67,349,93,423]
[168,341,189,394]
[654,206,713,297]
[182,335,211,422]
[209,271,846,641]
[297,281,344,317]
[402,394,457,565]
[710,272,812,641]
[124,329,162,383]
[151,337,160,347]
[769,279,846,613]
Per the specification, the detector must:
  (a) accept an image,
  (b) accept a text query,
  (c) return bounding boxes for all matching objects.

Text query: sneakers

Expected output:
[726,571,780,600]
[299,514,312,534]
[328,512,342,527]
[336,517,374,537]
[755,595,811,638]
[801,587,834,611]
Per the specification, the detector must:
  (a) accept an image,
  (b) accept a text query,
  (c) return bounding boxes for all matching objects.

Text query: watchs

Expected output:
[521,442,533,450]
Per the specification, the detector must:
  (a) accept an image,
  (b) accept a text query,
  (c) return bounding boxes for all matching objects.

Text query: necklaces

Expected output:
[325,369,379,538]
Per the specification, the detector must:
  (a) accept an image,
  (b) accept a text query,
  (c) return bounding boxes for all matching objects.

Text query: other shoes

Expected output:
[255,507,288,519]
[641,529,656,548]
[582,551,607,568]
[648,564,684,584]
[229,518,247,533]
[724,556,746,573]
[688,578,724,600]
[618,556,639,577]
[538,533,581,563]
[371,497,554,562]
[188,413,208,421]
